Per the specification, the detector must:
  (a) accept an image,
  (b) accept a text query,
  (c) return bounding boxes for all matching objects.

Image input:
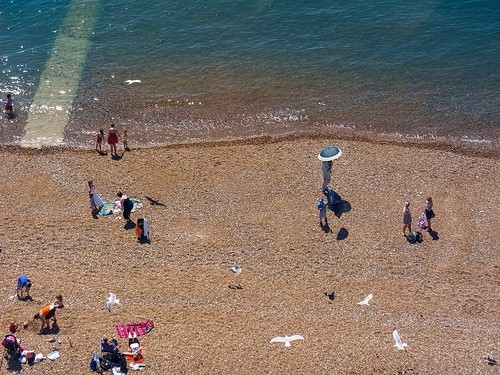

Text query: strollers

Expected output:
[2,335,21,360]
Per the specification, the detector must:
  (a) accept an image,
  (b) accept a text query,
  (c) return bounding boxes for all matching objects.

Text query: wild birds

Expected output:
[392,329,409,351]
[358,294,373,307]
[269,333,304,347]
[106,290,120,312]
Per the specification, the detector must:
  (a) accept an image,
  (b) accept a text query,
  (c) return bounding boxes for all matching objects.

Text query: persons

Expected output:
[5,94,14,114]
[95,128,105,152]
[107,124,120,156]
[90,328,142,375]
[403,201,412,235]
[322,187,341,207]
[16,275,32,299]
[2,321,36,370]
[321,160,333,192]
[317,198,327,225]
[34,305,57,332]
[122,129,128,149]
[425,197,435,231]
[117,191,133,227]
[88,180,98,212]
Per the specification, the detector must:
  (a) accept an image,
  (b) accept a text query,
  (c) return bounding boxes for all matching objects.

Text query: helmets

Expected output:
[319,197,323,202]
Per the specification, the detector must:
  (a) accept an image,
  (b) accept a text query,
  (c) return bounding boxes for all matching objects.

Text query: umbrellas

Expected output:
[317,146,342,161]
